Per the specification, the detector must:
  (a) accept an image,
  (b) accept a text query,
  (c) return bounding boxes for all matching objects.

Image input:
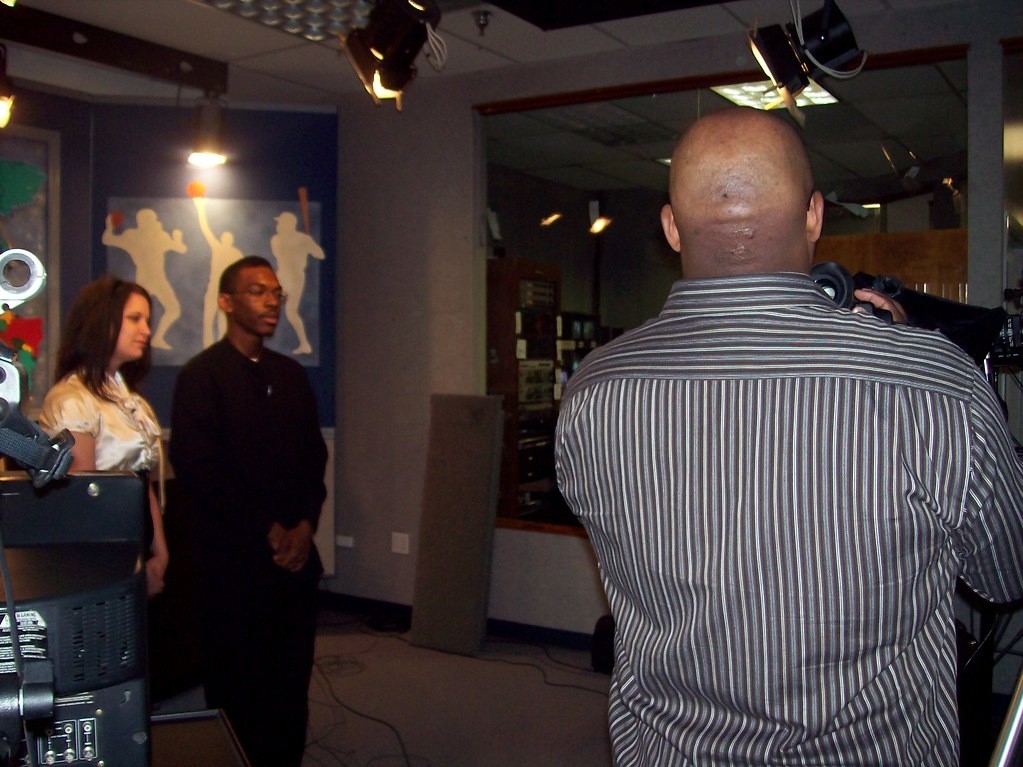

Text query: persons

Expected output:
[168,254,329,766]
[37,276,171,599]
[551,105,1023,766]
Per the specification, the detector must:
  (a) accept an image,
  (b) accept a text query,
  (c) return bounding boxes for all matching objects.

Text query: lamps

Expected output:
[744,1,868,102]
[333,0,454,112]
[186,90,233,170]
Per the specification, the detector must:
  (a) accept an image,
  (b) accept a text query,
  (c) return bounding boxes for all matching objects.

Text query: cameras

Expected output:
[810,262,902,326]
